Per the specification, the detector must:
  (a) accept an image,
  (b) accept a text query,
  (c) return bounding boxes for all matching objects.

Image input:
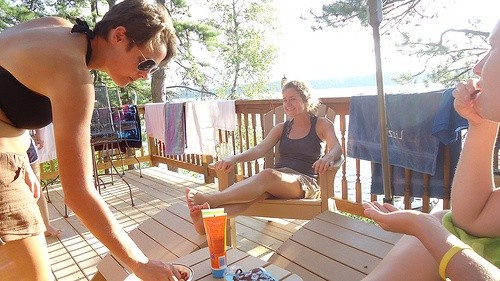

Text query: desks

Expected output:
[171,243,303,281]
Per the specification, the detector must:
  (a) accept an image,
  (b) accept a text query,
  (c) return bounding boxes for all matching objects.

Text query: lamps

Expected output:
[281,76,286,93]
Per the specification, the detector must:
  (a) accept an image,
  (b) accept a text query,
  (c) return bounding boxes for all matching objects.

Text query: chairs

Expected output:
[207,102,345,247]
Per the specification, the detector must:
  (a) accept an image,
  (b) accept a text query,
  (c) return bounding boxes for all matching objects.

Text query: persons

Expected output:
[358,16,500,281]
[0,0,181,281]
[186,80,342,235]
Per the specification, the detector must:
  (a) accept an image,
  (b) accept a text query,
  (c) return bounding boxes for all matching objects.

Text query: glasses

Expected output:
[132,40,159,74]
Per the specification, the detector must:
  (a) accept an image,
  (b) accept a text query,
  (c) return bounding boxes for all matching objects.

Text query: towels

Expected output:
[145,103,166,147]
[430,88,479,144]
[90,105,142,152]
[183,101,216,157]
[165,101,185,155]
[346,91,463,200]
[212,100,237,132]
[28,121,59,164]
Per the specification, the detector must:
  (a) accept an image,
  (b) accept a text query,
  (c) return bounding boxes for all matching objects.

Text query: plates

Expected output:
[124,263,195,281]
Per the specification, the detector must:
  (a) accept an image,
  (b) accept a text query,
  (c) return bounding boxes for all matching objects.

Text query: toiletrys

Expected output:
[199,207,228,279]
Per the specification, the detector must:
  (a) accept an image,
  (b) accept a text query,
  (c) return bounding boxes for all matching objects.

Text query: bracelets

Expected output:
[439,243,473,281]
